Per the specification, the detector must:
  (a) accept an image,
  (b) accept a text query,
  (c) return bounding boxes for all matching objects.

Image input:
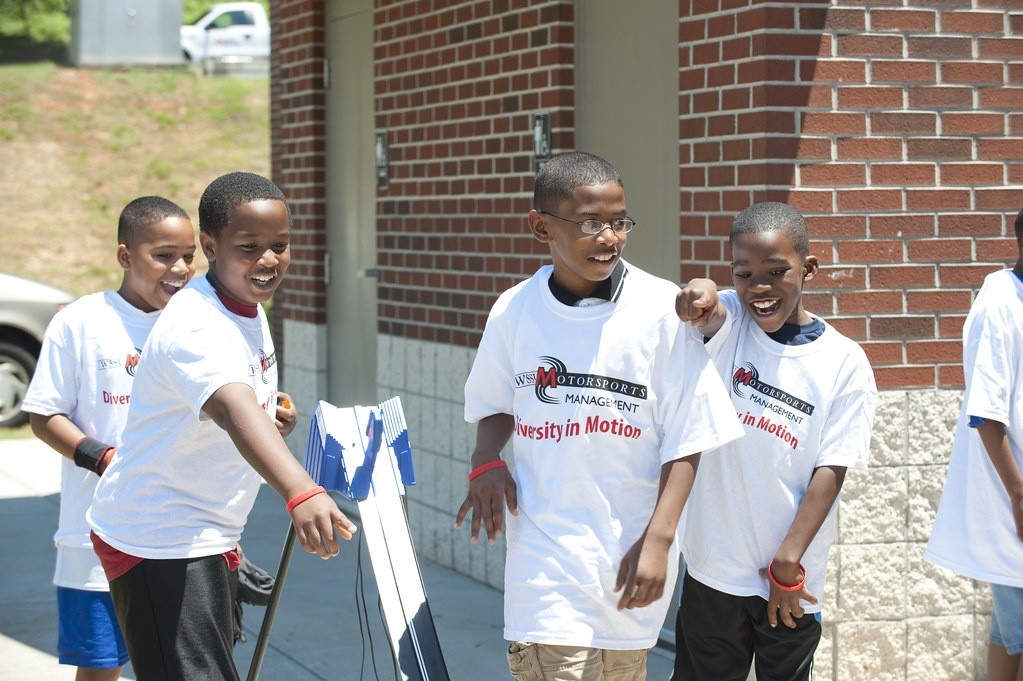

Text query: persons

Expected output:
[923,209,1023,681]
[21,196,295,680]
[453,150,746,681]
[665,200,877,681]
[85,174,357,680]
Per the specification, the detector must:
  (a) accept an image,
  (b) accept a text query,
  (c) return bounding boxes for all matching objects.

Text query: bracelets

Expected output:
[469,459,507,481]
[285,486,326,513]
[74,436,116,476]
[767,560,806,592]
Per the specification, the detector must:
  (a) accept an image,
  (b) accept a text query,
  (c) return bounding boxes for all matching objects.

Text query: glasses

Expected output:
[537,209,635,235]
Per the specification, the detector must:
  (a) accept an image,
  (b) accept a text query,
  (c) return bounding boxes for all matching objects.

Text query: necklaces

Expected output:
[610,263,624,303]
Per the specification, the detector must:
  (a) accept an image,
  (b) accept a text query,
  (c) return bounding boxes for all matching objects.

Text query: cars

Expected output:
[0,272,73,431]
[178,1,273,62]
